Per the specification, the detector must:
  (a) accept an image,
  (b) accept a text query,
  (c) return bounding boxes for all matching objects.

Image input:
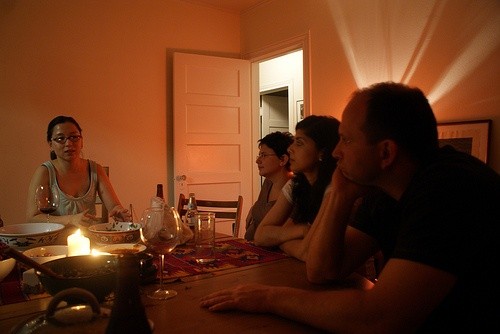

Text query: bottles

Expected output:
[103,254,154,334]
[155,184,165,202]
[184,193,199,242]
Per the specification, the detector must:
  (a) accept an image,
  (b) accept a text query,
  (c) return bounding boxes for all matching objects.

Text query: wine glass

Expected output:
[140,207,181,300]
[34,184,60,223]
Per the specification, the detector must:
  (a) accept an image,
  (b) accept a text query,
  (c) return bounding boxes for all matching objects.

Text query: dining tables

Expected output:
[0,232,375,334]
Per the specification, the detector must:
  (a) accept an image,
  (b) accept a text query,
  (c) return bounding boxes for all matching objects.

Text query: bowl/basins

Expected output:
[0,223,66,253]
[95,243,148,269]
[87,221,141,245]
[22,245,67,264]
[137,253,154,273]
[34,256,142,306]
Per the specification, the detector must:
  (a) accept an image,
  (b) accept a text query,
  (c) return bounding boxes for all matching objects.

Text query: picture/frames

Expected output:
[436,119,492,165]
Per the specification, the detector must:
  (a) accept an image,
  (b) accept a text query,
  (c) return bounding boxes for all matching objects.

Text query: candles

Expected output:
[68,228,90,258]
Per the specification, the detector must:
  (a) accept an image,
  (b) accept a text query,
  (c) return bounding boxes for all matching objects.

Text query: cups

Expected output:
[195,212,216,263]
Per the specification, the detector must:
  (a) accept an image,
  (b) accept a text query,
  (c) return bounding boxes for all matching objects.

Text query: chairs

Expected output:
[95,166,110,223]
[177,194,243,238]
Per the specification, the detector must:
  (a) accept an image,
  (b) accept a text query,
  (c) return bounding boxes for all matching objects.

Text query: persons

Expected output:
[25,115,133,229]
[243,131,296,243]
[199,81,500,334]
[253,115,378,272]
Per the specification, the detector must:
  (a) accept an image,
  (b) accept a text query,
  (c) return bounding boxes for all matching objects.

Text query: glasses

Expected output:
[50,135,83,144]
[256,152,276,159]
